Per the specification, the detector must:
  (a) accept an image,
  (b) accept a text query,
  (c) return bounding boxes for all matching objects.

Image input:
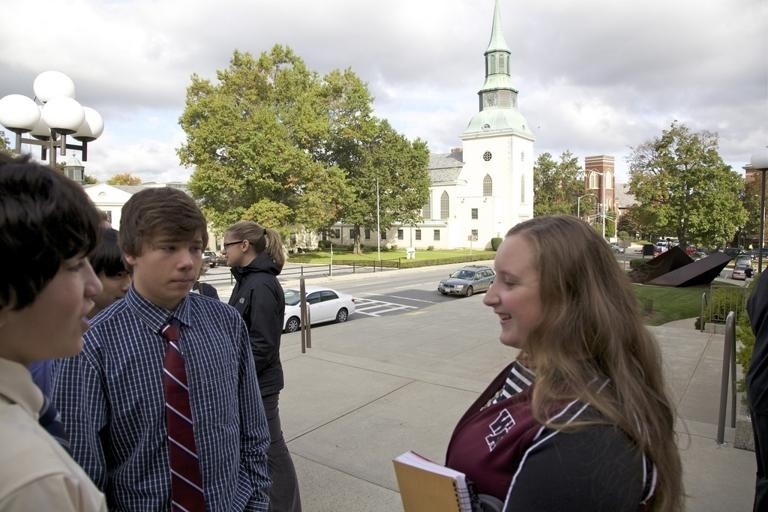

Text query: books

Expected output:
[392,448,482,512]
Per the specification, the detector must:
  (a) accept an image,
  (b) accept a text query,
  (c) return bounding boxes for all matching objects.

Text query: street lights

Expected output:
[750,148,768,274]
[577,194,594,218]
[0,70,104,169]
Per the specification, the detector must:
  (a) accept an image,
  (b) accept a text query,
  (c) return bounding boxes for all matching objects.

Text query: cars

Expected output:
[610,244,624,254]
[438,265,497,298]
[643,239,670,258]
[732,248,768,281]
[201,251,229,267]
[282,286,356,333]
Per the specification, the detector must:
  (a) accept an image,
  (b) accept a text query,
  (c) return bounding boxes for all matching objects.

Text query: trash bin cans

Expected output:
[643,244,654,259]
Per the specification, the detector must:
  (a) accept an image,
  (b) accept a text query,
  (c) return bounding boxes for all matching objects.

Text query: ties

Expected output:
[160,316,209,512]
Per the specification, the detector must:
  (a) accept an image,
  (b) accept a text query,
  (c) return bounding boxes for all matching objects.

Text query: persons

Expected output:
[223,223,301,509]
[745,262,768,510]
[46,186,271,511]
[442,214,686,510]
[1,152,111,512]
[30,224,130,439]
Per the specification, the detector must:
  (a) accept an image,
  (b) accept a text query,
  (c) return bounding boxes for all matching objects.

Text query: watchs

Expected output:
[189,280,222,300]
